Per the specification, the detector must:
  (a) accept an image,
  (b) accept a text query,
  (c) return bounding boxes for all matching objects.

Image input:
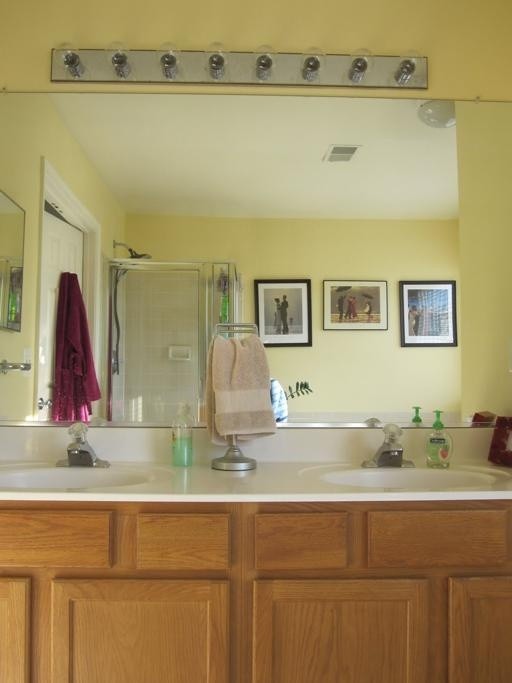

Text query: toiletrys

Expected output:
[410,406,424,422]
[424,410,455,471]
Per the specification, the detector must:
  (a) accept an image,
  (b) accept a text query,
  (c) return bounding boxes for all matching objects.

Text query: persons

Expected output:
[409,304,421,336]
[362,296,373,319]
[280,294,289,334]
[273,296,282,334]
[343,294,352,319]
[335,292,346,321]
[351,295,358,317]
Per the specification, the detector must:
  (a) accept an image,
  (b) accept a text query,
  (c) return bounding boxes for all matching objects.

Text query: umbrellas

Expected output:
[334,285,352,294]
[361,291,374,299]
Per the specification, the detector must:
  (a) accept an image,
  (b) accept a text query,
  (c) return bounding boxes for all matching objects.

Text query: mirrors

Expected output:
[0,190,24,331]
[0,92,511,428]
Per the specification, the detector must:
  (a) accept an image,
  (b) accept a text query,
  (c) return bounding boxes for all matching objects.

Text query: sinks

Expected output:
[0,464,179,490]
[296,464,511,491]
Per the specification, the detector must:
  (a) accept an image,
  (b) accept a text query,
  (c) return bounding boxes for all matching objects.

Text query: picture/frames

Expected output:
[252,278,457,346]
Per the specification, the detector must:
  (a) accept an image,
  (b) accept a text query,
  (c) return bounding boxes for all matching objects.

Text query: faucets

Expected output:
[366,418,379,423]
[361,423,416,469]
[56,422,111,469]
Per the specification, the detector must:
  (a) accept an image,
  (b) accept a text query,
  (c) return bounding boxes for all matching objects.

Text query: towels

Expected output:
[206,332,276,445]
